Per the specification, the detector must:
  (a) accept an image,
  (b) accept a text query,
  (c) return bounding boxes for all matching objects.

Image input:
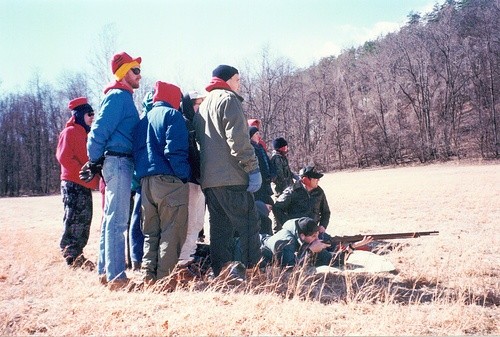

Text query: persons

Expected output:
[134,64,331,289]
[196,215,375,269]
[76,52,155,293]
[55,96,99,273]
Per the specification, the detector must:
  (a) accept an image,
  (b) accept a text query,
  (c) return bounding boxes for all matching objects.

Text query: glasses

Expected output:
[130,67,140,75]
[85,112,94,117]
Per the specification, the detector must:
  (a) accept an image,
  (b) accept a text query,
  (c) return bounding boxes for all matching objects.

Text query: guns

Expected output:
[297,230,439,253]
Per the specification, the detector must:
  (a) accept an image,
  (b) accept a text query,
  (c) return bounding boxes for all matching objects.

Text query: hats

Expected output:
[256,194,274,206]
[71,105,94,134]
[249,126,259,138]
[68,97,88,111]
[212,65,238,83]
[272,137,287,150]
[297,217,321,235]
[189,91,207,99]
[299,166,324,178]
[115,61,139,82]
[111,52,142,74]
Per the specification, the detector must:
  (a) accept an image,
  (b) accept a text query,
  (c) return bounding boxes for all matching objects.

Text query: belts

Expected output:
[104,151,133,158]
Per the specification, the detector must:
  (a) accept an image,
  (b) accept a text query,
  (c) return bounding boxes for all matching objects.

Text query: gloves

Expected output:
[78,160,99,183]
[246,171,262,193]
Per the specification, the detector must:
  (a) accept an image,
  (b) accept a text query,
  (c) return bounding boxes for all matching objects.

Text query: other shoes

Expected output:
[156,276,184,292]
[71,258,95,271]
[143,278,155,288]
[106,278,142,291]
[176,263,202,282]
[99,274,108,287]
